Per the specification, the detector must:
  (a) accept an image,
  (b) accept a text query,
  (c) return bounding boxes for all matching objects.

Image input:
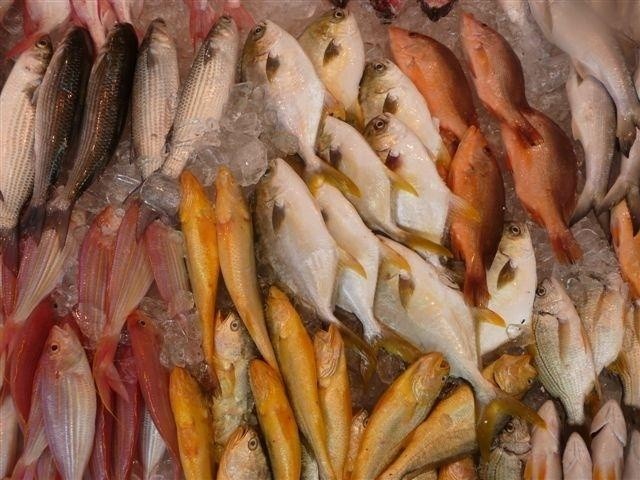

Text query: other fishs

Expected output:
[1,0,639,480]
